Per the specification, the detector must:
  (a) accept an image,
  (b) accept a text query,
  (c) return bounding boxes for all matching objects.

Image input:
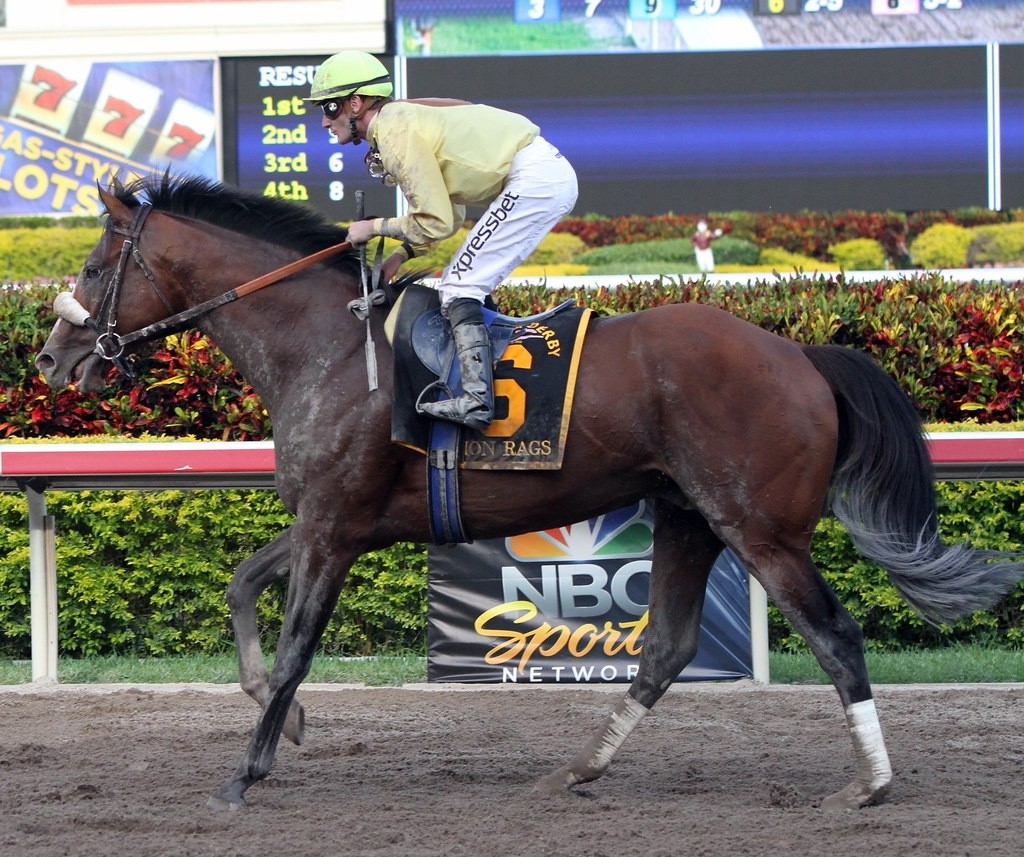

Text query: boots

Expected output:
[419,298,493,430]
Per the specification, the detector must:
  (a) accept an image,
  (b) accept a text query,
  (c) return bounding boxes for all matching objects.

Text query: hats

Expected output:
[303,49,394,103]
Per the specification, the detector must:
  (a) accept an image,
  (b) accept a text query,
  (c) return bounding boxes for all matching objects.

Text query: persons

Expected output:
[304,53,580,427]
[692,222,721,272]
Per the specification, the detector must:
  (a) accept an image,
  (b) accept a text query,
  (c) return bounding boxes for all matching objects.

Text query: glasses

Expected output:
[321,96,348,119]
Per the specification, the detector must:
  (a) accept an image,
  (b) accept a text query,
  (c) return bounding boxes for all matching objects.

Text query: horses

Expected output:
[30,160,1024,813]
